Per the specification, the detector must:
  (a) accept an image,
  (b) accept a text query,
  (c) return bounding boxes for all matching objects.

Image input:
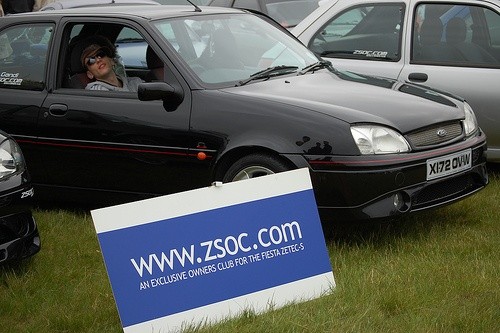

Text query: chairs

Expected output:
[418,18,468,65]
[65,34,126,90]
[146,44,166,80]
[443,17,498,65]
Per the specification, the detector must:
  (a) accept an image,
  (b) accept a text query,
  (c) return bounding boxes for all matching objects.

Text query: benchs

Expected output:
[315,33,398,61]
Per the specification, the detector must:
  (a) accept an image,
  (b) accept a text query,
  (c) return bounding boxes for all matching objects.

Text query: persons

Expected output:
[79,43,143,93]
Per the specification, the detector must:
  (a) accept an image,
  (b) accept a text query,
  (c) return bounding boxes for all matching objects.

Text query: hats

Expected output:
[81,44,102,67]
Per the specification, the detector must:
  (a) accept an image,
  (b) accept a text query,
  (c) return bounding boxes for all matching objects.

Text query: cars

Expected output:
[17,0,500,167]
[0,130,41,263]
[0,0,491,221]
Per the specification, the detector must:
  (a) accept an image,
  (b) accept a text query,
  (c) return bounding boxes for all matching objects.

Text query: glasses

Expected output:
[85,50,106,66]
